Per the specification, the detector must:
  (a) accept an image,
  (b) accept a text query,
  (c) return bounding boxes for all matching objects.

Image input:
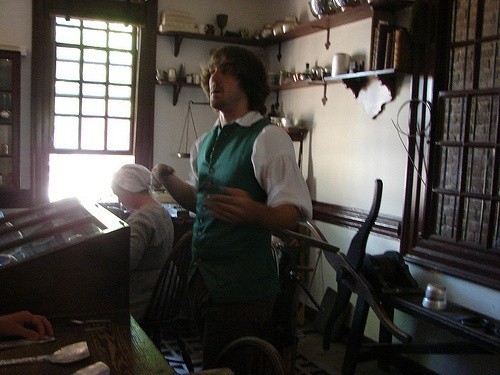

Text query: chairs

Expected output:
[139,230,196,373]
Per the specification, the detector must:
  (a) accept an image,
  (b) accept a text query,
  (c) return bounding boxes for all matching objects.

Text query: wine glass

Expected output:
[216,13,228,36]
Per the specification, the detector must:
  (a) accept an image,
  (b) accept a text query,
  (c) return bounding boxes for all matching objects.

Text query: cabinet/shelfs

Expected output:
[155,0,410,90]
[0,49,21,194]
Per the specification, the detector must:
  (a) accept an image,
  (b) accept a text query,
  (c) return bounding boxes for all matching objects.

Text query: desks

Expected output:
[0,312,178,375]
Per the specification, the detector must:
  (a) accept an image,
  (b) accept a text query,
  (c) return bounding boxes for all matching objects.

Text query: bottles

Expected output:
[300,62,313,82]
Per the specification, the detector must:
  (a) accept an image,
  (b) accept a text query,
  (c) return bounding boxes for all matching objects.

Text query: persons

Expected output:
[0,311,55,340]
[113,164,175,322]
[151,45,313,368]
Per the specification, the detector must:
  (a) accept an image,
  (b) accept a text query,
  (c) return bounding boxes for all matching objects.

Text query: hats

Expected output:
[115,164,154,193]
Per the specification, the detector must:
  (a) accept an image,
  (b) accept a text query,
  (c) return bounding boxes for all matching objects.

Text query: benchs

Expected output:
[342,288,500,375]
